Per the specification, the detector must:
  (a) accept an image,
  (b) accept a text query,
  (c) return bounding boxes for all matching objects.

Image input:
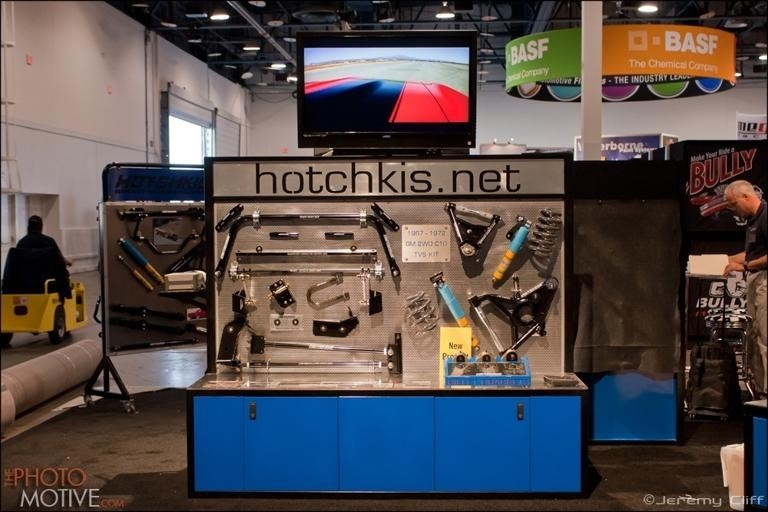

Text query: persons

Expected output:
[16,214,72,294]
[724,180,768,401]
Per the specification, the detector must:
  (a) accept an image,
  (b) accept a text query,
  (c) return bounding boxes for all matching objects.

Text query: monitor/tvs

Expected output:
[296,29,477,157]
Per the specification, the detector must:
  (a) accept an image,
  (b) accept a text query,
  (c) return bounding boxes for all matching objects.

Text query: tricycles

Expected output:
[0,278,90,349]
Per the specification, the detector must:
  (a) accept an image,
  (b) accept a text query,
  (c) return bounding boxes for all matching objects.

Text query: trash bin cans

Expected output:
[743,399,768,512]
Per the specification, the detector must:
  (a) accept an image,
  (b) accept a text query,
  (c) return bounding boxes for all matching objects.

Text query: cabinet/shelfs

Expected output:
[186,373,593,499]
